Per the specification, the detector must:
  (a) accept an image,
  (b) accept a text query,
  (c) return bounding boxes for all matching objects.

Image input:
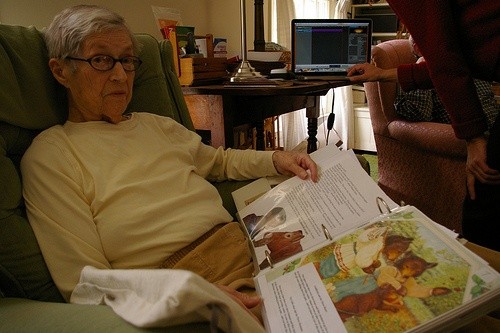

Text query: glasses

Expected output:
[68,53,143,72]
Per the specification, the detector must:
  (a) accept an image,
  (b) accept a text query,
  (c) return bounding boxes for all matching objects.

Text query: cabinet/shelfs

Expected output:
[169,32,227,87]
[352,3,412,152]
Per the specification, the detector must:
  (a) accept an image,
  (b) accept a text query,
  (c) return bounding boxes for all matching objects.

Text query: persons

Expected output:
[19,3,318,325]
[346,0,500,252]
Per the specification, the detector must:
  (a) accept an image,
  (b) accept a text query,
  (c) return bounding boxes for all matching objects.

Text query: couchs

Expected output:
[364,39,500,238]
[0,24,370,333]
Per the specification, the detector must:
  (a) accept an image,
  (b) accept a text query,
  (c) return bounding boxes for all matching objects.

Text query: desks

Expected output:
[182,80,367,155]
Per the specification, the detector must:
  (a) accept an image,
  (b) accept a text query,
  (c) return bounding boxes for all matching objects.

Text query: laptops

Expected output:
[291,18,373,80]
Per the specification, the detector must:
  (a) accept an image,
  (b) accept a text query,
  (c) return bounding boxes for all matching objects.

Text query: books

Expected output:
[231,143,500,333]
